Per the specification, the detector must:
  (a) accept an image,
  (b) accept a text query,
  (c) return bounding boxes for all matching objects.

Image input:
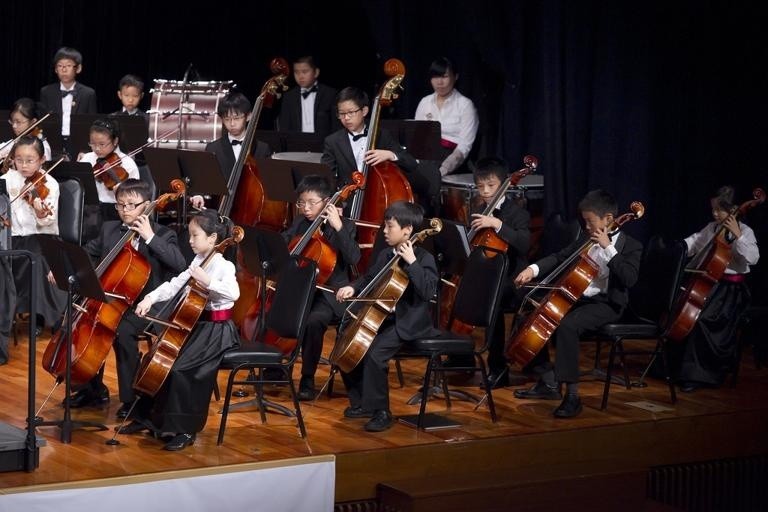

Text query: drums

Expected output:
[441,171,543,313]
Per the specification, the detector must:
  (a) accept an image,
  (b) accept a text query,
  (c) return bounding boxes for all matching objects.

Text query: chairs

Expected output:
[705,269,758,387]
[536,209,564,262]
[407,244,509,426]
[216,253,319,446]
[165,221,195,272]
[59,176,85,246]
[593,228,689,412]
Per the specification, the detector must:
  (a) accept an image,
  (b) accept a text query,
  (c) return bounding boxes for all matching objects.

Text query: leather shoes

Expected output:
[344,408,375,417]
[247,371,291,385]
[480,371,509,388]
[298,376,314,400]
[365,410,392,431]
[440,361,476,375]
[515,381,561,400]
[555,394,582,417]
[63,384,195,451]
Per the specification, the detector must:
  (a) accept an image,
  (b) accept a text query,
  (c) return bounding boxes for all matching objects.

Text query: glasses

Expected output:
[88,142,112,149]
[296,198,324,209]
[8,119,31,125]
[336,108,362,119]
[55,63,73,70]
[115,200,146,211]
[14,158,42,165]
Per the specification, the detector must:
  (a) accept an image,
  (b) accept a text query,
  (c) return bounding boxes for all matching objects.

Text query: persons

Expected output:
[47,177,192,419]
[438,155,533,390]
[333,200,439,432]
[513,188,644,421]
[245,173,361,403]
[0,46,480,333]
[116,208,242,453]
[677,183,760,394]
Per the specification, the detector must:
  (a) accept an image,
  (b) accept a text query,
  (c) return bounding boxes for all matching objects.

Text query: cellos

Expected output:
[346,59,414,272]
[132,226,244,397]
[436,156,543,336]
[661,188,765,339]
[242,172,367,356]
[216,54,292,344]
[328,219,443,374]
[503,201,644,366]
[42,178,186,387]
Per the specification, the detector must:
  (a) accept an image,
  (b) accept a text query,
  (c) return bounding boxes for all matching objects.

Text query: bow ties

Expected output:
[493,208,500,217]
[231,140,243,144]
[61,90,78,97]
[302,85,317,98]
[354,128,367,141]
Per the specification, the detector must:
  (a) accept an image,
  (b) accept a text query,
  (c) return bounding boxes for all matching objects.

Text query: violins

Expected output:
[93,152,129,191]
[22,170,54,218]
[2,122,39,176]
